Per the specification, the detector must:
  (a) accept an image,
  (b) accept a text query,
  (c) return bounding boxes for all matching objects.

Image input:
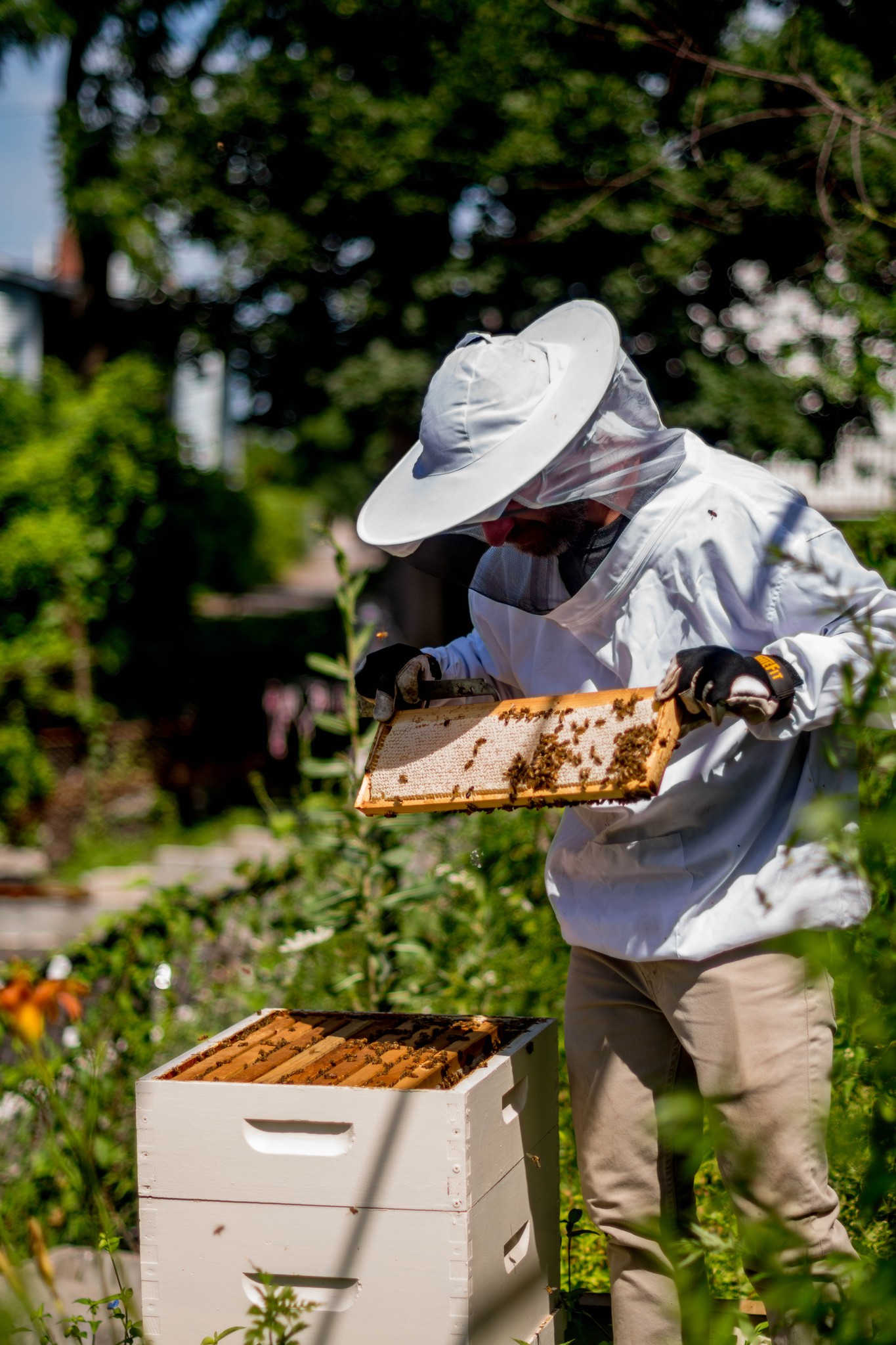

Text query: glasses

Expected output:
[500,471,549,518]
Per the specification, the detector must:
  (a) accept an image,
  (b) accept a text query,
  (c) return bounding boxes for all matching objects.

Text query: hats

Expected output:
[355,299,622,548]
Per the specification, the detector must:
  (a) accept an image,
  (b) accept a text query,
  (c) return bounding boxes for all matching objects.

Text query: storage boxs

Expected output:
[135,1007,567,1345]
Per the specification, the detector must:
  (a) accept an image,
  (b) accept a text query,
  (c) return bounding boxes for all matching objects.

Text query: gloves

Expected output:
[354,643,442,724]
[654,645,803,737]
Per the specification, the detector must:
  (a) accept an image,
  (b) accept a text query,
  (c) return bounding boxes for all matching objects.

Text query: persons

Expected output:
[354,295,896,1345]
